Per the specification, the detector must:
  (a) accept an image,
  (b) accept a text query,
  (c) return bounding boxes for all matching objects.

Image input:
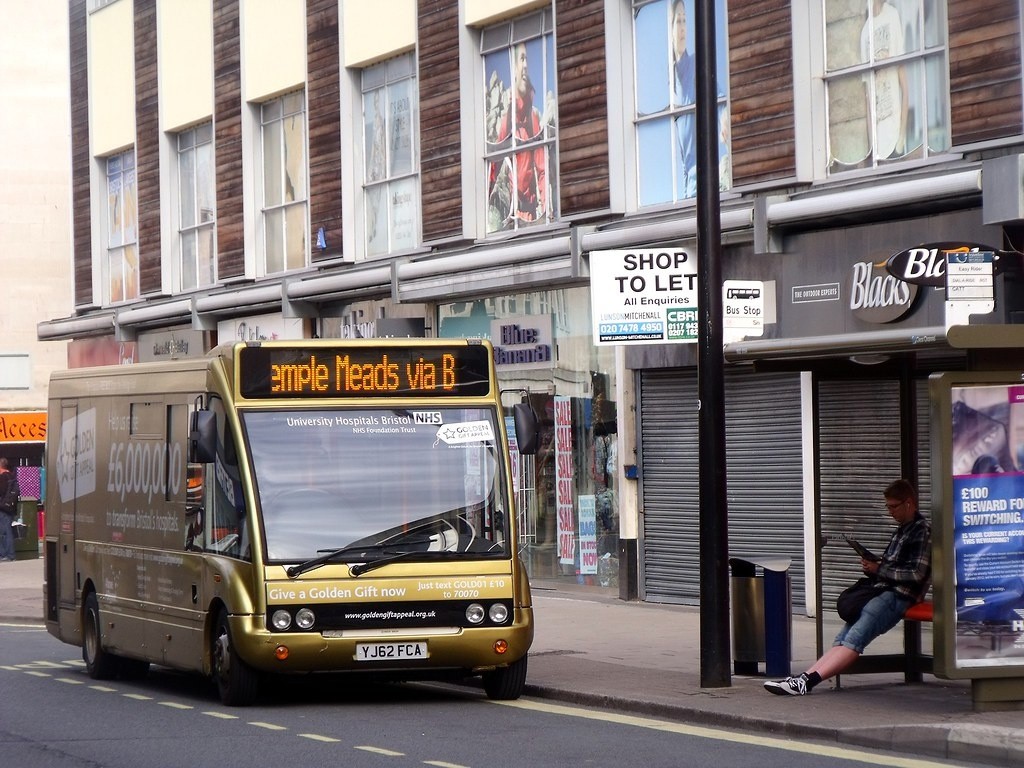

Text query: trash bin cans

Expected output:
[13,496,39,562]
[728,556,793,680]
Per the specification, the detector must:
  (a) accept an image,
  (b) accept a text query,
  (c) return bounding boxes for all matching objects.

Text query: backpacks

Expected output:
[0,473,21,516]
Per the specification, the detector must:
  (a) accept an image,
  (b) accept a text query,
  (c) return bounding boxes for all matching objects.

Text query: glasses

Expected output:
[885,500,906,511]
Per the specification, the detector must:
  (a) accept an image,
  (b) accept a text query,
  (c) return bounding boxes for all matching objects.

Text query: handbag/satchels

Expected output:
[837,578,888,625]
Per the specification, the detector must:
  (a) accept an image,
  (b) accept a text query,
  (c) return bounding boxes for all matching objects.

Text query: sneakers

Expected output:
[776,674,812,696]
[764,675,801,695]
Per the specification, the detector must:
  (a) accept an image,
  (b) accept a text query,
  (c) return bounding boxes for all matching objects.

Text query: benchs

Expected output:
[906,601,933,622]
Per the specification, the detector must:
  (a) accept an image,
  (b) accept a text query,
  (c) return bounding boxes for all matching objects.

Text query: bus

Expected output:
[38,338,545,707]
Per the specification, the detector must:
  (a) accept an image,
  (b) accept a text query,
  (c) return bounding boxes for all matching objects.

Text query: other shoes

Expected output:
[0,555,16,561]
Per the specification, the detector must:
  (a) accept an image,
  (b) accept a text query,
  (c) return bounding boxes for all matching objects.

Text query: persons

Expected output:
[761,479,932,696]
[0,458,20,562]
[487,42,554,229]
[367,89,384,243]
[667,0,729,199]
[861,0,909,161]
